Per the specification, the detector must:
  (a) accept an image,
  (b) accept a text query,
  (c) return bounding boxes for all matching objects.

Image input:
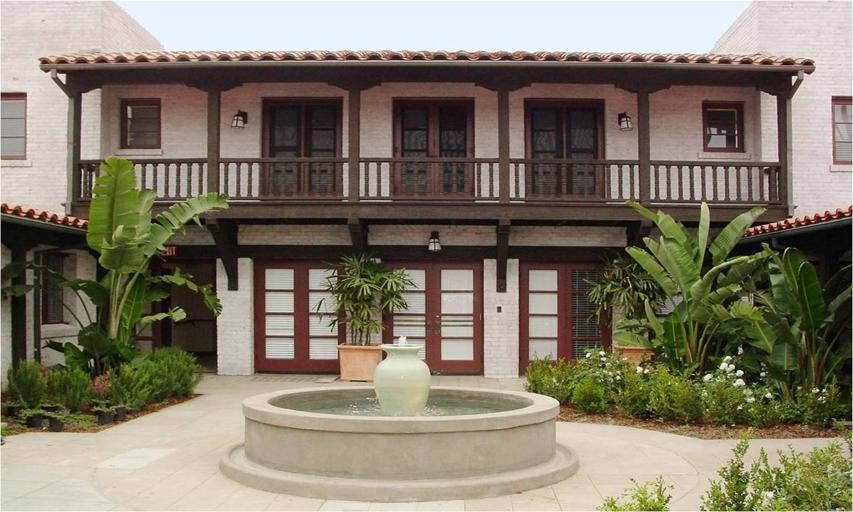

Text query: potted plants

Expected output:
[583,250,668,371]
[311,249,418,382]
[3,399,126,432]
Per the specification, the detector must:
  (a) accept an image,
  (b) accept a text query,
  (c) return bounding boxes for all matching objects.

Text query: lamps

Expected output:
[231,112,248,129]
[617,113,634,131]
[427,232,442,253]
